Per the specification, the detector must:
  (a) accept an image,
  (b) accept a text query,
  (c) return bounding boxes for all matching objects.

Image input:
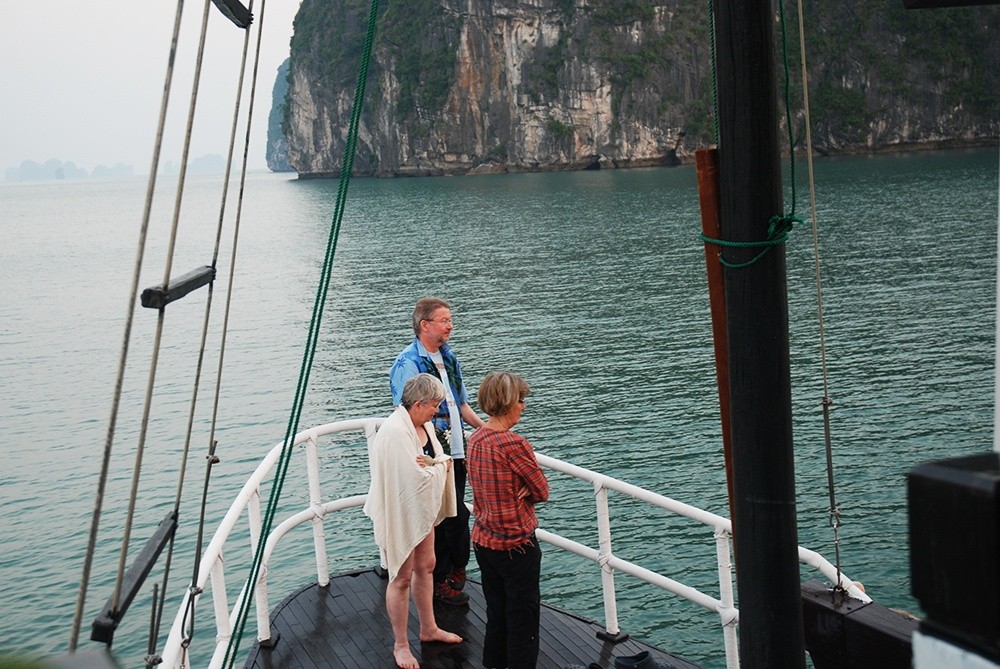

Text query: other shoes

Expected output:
[432,579,472,606]
[449,569,467,590]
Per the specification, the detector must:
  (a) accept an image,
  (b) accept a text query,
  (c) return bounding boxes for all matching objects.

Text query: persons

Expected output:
[389,298,486,605]
[362,373,462,669]
[466,373,549,669]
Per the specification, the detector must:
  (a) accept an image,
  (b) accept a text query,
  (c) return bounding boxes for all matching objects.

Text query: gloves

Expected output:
[432,416,449,433]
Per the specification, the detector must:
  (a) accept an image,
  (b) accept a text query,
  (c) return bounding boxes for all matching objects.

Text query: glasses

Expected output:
[419,317,453,324]
[518,400,526,404]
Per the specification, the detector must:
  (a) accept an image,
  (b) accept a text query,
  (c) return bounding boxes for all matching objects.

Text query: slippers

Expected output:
[614,650,673,669]
